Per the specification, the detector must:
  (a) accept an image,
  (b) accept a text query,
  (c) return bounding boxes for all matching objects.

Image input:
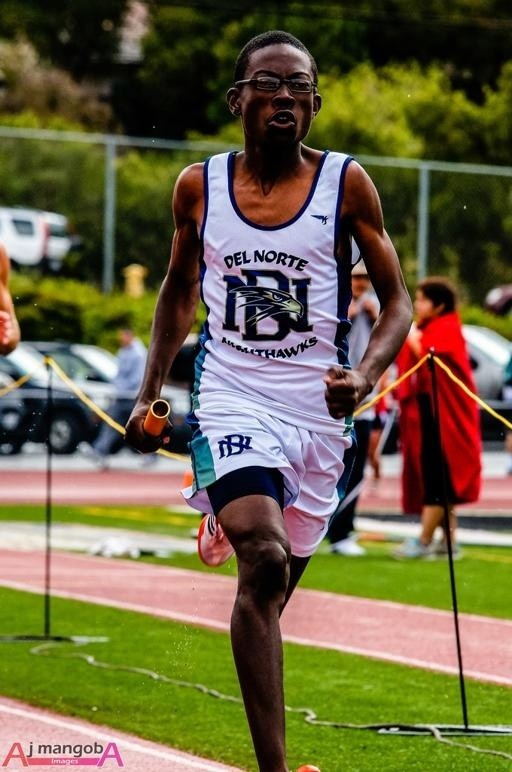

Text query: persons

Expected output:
[325,260,383,556]
[78,323,150,472]
[0,237,22,358]
[361,362,399,490]
[124,31,413,771]
[388,276,481,563]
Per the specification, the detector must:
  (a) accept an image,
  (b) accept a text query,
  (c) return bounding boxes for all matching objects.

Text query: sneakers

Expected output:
[392,536,466,563]
[197,513,236,568]
[330,538,366,558]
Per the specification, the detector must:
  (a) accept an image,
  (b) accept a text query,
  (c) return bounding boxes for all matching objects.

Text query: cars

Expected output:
[1,331,204,453]
[0,206,75,274]
[349,319,512,433]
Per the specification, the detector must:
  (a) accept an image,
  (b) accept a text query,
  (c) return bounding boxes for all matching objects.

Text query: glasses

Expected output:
[234,76,317,94]
